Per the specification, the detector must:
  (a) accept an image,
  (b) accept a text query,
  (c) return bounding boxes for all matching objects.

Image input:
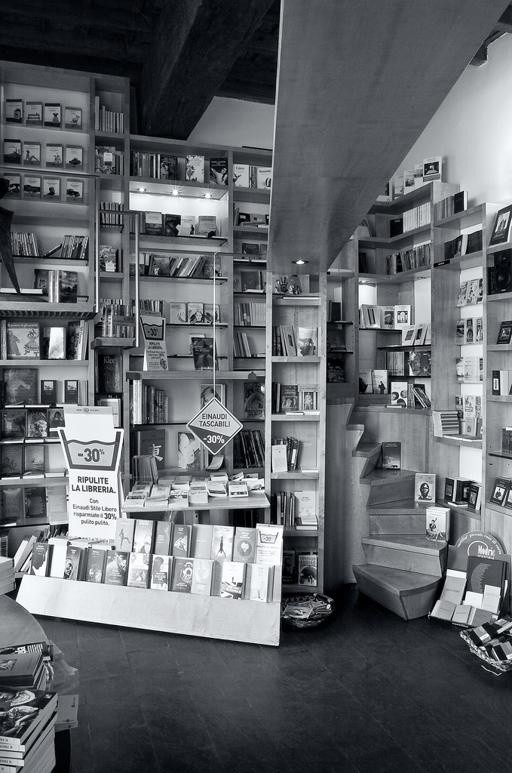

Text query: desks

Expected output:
[0,595,71,772]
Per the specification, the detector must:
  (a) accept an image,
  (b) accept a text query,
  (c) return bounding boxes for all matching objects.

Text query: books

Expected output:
[0,641,58,772]
[326,156,512,628]
[270,273,317,586]
[1,98,125,361]
[122,152,273,371]
[1,366,283,599]
